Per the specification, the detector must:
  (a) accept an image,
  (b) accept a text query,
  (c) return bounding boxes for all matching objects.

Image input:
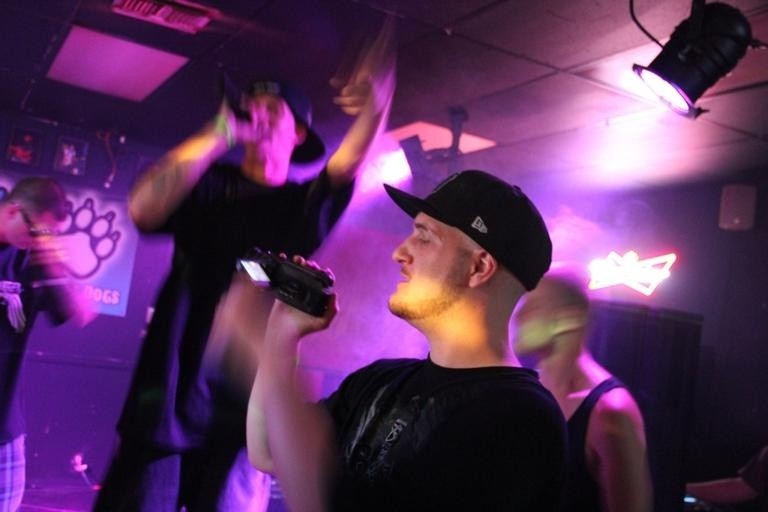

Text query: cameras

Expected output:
[234,244,332,318]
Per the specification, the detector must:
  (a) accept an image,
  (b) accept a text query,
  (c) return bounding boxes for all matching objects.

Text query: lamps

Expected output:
[399,105,472,184]
[631,2,768,122]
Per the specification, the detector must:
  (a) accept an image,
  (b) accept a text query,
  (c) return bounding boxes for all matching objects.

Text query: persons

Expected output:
[242,170,603,512]
[1,177,103,511]
[86,30,406,511]
[684,442,768,504]
[512,268,659,511]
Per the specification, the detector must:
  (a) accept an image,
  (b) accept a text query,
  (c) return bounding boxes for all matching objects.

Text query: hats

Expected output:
[246,73,326,163]
[384,170,552,291]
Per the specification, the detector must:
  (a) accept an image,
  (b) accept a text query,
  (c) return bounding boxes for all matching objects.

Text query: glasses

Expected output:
[20,210,51,237]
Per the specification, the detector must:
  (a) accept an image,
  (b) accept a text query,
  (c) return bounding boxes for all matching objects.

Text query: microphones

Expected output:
[217,70,253,123]
